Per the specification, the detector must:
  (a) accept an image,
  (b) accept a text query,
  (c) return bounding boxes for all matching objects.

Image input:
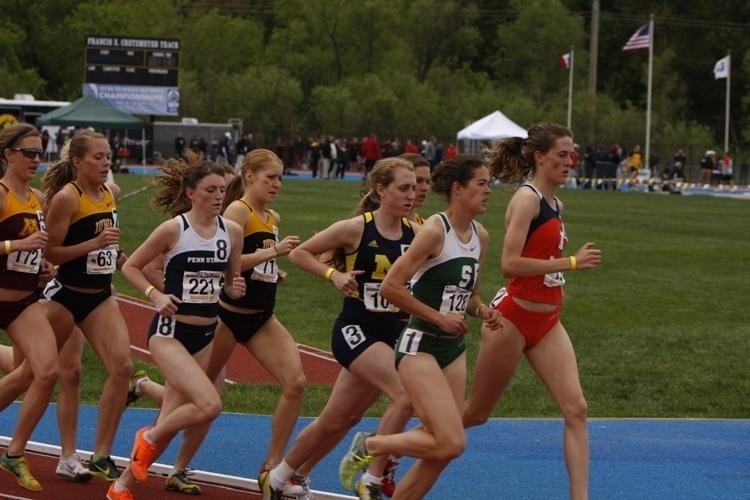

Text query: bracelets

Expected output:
[474,303,486,316]
[323,267,335,279]
[570,256,578,269]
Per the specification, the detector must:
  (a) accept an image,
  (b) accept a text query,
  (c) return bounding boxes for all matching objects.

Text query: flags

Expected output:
[559,51,571,68]
[621,22,649,50]
[712,54,729,81]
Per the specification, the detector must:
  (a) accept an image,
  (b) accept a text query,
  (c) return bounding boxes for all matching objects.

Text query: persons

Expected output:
[362,152,432,497]
[0,116,133,491]
[570,144,688,183]
[176,131,302,176]
[480,140,494,174]
[258,156,423,500]
[166,148,304,498]
[382,121,602,500]
[313,135,457,179]
[699,150,735,185]
[106,149,247,499]
[339,151,507,499]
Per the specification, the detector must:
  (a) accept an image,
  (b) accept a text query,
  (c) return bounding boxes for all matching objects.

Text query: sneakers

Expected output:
[1,369,201,500]
[259,431,395,500]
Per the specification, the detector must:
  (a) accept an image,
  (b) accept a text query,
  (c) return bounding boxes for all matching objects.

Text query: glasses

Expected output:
[11,148,44,159]
